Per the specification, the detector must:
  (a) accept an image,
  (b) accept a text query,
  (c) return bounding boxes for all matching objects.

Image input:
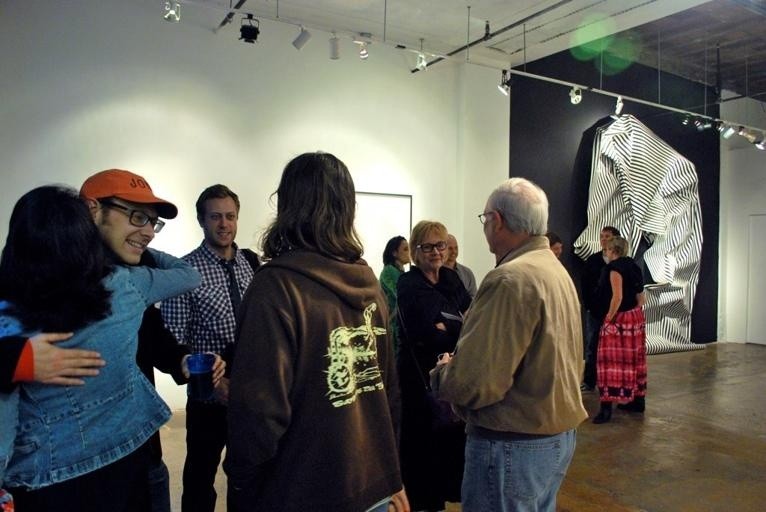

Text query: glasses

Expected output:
[111,203,165,233]
[416,241,446,252]
[478,212,491,224]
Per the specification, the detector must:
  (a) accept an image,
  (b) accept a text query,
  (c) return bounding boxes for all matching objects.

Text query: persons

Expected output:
[396,219,471,512]
[0,167,228,512]
[230,151,409,512]
[547,223,649,425]
[429,175,589,512]
[380,234,415,428]
[0,185,204,511]
[441,233,480,303]
[160,181,270,512]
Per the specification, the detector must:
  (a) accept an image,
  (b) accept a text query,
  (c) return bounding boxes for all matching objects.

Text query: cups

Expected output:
[185,353,216,400]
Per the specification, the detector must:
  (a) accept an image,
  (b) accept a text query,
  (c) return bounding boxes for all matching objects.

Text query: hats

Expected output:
[79,169,177,218]
